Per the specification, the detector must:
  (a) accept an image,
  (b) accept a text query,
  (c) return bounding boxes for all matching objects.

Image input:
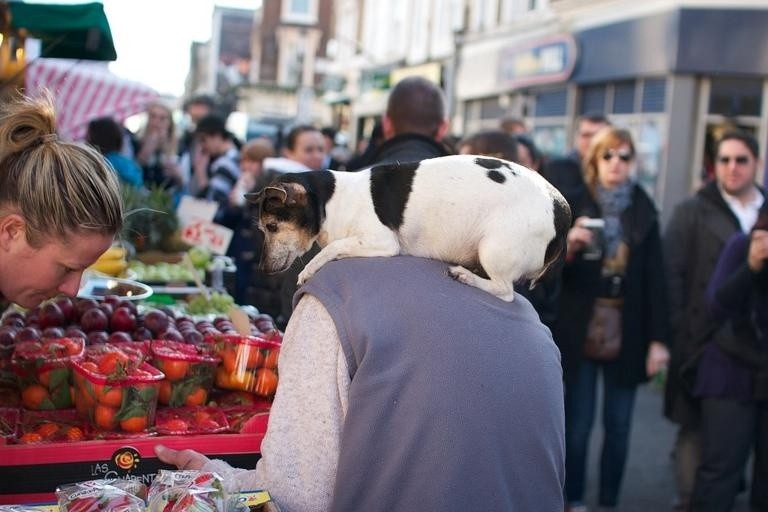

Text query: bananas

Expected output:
[89,247,130,279]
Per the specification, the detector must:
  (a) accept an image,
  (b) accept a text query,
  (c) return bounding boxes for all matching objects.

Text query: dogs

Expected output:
[243,153,572,304]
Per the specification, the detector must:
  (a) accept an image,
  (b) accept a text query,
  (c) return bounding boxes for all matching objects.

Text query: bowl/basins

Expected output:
[77,274,154,306]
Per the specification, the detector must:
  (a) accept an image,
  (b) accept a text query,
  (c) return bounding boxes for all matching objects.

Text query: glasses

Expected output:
[719,155,748,163]
[602,148,632,161]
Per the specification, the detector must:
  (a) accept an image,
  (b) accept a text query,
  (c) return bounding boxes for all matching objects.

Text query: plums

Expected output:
[0,294,277,346]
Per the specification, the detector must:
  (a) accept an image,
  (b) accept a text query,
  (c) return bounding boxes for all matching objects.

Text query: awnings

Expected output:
[25,59,174,144]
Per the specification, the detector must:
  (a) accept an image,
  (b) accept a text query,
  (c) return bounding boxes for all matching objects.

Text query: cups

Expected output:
[581,217,606,259]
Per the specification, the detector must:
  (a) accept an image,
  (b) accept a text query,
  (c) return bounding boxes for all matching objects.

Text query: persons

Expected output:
[458,112,768,511]
[0,93,126,309]
[87,106,183,188]
[154,76,566,511]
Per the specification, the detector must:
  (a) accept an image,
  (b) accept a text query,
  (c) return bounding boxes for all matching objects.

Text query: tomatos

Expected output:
[0,336,280,446]
